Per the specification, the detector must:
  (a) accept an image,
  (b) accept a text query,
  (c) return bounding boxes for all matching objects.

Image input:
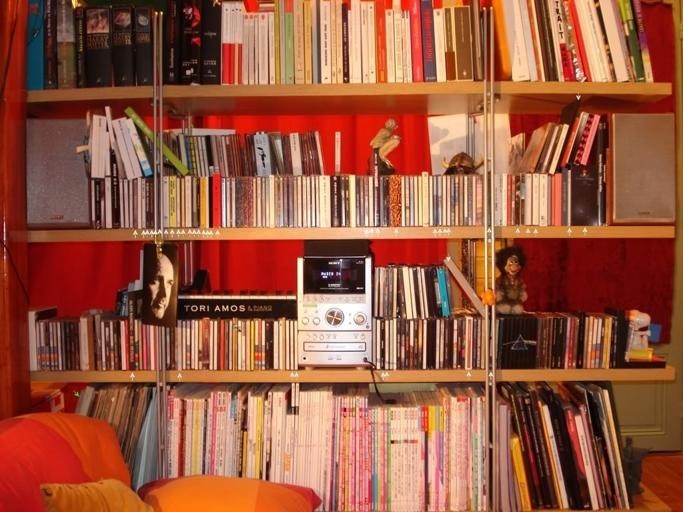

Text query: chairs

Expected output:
[0,412,323,512]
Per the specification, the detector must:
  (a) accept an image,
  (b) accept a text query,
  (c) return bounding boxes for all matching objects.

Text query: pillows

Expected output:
[39,479,154,512]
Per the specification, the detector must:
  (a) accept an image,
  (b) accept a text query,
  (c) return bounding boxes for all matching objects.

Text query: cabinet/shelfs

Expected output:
[0,0,683,512]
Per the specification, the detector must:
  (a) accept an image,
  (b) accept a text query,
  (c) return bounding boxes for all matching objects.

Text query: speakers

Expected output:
[26,119,92,229]
[610,112,675,225]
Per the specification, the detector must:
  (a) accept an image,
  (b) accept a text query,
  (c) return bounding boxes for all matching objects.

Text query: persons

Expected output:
[623,436,649,492]
[495,244,528,314]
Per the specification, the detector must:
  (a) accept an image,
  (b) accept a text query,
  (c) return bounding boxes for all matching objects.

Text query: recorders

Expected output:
[297,255,375,370]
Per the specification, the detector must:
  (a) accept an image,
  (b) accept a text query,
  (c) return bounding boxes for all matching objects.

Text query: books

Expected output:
[25,0,655,90]
[77,107,610,229]
[29,257,488,372]
[489,309,628,369]
[74,383,632,510]
